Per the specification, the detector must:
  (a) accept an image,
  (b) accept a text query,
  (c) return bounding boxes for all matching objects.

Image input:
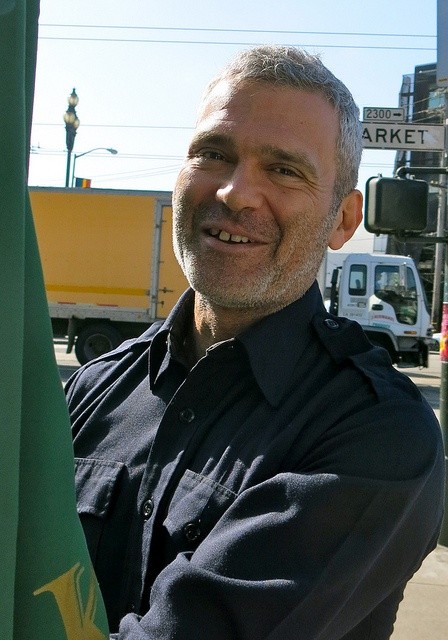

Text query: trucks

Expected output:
[27,187,433,369]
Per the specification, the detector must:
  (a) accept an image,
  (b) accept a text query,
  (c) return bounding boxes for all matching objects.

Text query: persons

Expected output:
[64,46,447,638]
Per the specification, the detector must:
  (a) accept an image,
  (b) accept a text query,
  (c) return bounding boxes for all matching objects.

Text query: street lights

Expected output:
[73,147,118,187]
[64,89,79,186]
